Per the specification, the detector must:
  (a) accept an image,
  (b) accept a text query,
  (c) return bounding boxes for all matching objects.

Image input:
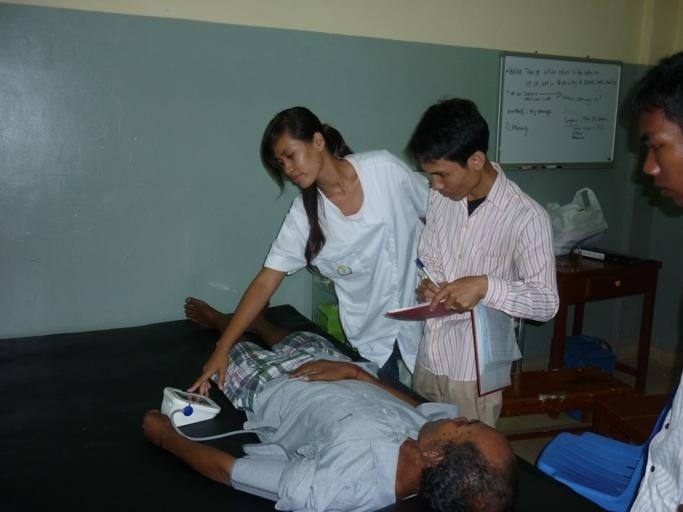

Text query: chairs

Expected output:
[537,403,671,511]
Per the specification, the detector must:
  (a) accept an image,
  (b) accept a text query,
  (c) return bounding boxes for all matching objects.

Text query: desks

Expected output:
[497,246,664,394]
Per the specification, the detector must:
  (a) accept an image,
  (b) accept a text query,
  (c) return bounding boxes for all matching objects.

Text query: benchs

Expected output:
[501,365,633,442]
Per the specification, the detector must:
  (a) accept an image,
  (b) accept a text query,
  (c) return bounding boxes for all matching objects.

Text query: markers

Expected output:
[519,164,564,169]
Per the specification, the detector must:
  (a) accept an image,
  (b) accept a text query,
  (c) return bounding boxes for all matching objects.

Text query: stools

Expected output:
[596,395,673,444]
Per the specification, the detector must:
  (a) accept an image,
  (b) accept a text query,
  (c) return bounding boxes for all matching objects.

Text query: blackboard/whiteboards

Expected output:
[496,51,623,170]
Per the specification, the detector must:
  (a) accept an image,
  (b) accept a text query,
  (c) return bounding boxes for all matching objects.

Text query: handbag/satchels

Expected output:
[562,335,615,420]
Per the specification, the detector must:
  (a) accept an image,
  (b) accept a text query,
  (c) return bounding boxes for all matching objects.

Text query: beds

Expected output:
[0,304,604,512]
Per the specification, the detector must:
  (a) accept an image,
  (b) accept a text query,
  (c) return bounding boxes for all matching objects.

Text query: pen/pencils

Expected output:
[416,258,440,289]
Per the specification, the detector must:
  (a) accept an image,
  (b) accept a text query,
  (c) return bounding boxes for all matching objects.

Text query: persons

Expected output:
[616,47,683,512]
[187,106,430,392]
[402,97,559,425]
[140,295,520,512]
